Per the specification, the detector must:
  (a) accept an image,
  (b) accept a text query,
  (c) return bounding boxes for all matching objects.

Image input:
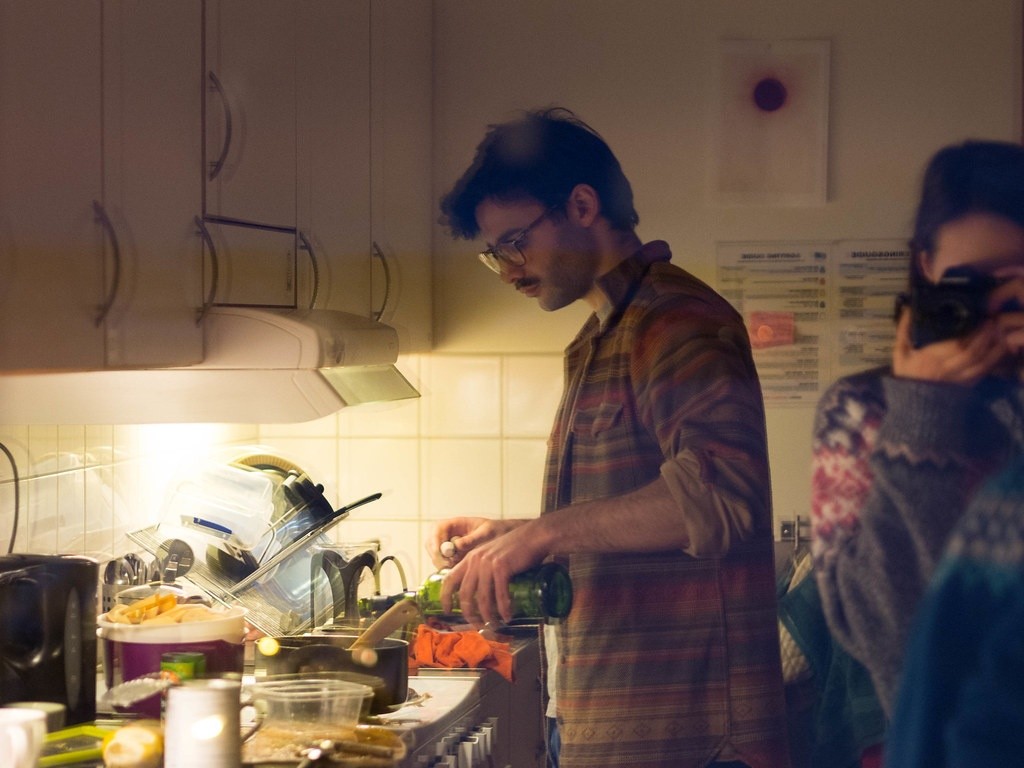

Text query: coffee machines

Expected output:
[0,555,100,725]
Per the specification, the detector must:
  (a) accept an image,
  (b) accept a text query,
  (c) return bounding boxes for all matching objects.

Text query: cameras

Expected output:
[914,264,1021,350]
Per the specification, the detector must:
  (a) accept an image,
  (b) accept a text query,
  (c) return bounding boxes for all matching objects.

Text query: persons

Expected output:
[428,110,793,768]
[811,139,1024,729]
[882,443,1024,767]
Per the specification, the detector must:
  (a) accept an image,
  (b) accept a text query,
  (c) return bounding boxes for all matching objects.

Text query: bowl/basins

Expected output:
[96,605,250,719]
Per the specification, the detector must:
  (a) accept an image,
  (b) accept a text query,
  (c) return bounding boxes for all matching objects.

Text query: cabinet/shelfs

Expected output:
[0,0,439,427]
[478,654,549,768]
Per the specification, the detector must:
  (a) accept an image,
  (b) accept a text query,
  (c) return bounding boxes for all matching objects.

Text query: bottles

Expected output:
[358,562,573,625]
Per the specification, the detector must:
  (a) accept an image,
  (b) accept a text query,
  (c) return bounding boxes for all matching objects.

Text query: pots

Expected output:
[255,635,410,714]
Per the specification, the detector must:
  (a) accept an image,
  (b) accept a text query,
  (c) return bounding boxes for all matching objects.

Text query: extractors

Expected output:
[1,306,422,424]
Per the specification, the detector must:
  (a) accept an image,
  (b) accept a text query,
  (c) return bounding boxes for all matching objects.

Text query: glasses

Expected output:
[479,205,558,274]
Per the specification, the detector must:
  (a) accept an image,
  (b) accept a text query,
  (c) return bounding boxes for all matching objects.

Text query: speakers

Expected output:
[0,553,99,729]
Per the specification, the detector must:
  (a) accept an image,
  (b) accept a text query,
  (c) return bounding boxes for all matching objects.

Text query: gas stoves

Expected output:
[96,674,498,768]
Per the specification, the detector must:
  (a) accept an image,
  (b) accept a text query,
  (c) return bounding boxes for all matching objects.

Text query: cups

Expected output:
[1,707,47,768]
[164,678,242,768]
[2,701,67,731]
